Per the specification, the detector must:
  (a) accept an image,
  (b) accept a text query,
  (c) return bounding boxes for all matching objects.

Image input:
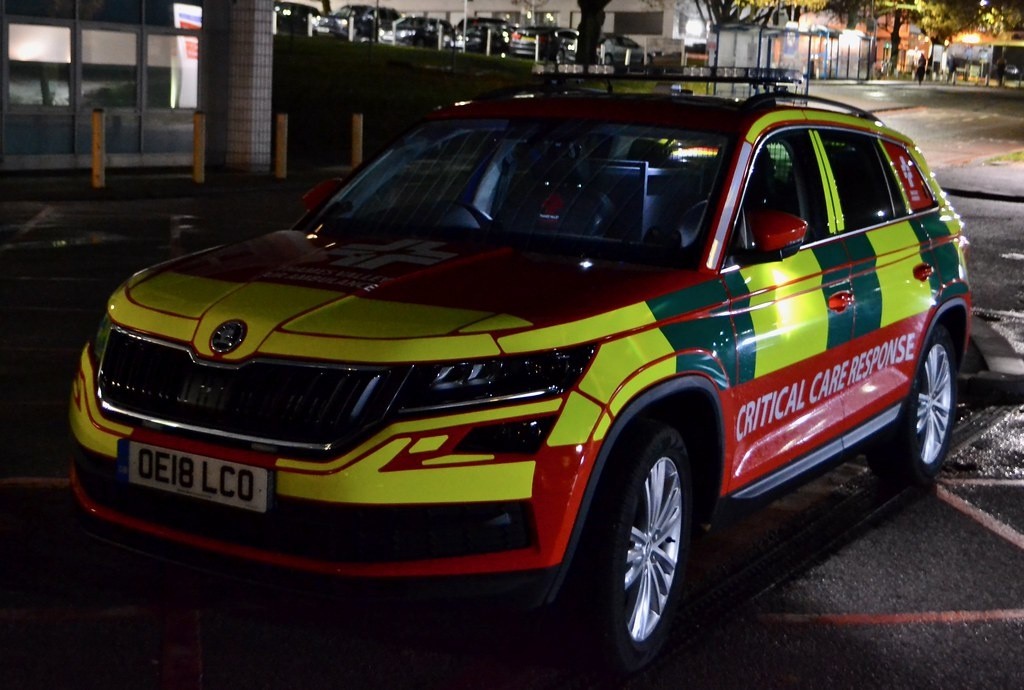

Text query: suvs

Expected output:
[67,68,973,678]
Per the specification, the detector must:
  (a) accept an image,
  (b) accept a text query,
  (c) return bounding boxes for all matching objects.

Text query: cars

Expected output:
[275,2,656,69]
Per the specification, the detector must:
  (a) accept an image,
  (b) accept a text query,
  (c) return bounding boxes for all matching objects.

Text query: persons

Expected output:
[914,55,926,81]
[946,55,956,86]
[996,53,1007,82]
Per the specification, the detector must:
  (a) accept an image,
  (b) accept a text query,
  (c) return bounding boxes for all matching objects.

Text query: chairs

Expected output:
[665,143,798,272]
[498,133,618,237]
